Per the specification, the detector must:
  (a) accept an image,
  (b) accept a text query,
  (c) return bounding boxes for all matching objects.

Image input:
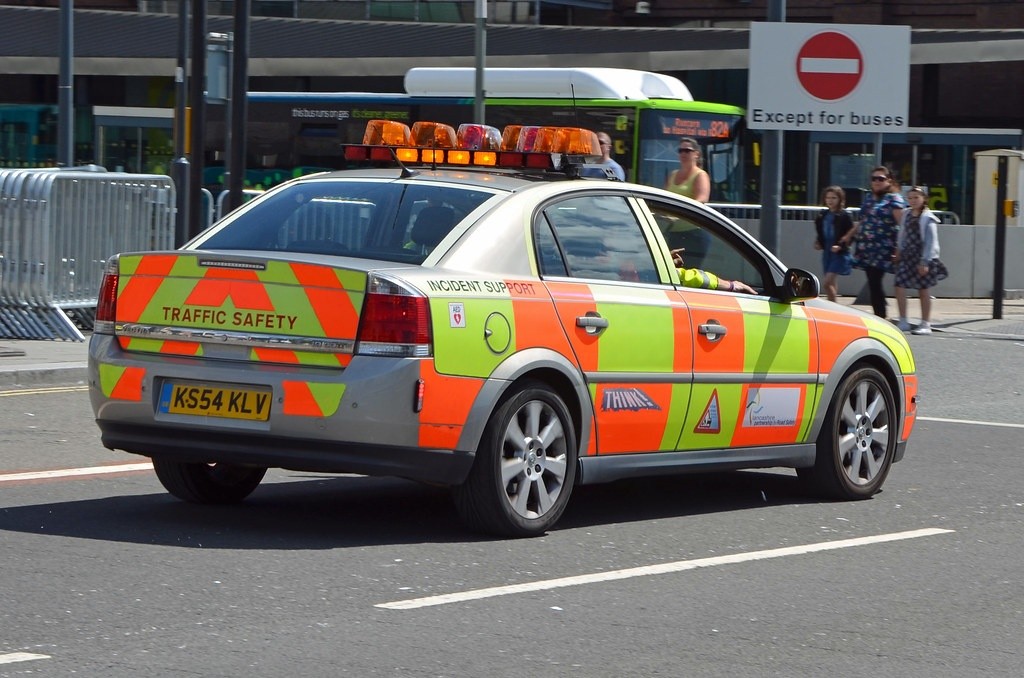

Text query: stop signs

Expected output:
[747,22,912,133]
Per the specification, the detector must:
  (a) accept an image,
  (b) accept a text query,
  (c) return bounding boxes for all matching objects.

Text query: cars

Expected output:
[87,120,919,538]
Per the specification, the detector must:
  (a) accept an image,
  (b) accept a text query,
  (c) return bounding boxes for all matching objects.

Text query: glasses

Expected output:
[871,175,888,182]
[677,148,697,154]
[598,140,611,145]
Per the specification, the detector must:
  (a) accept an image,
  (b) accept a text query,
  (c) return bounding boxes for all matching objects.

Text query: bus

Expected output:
[0,103,59,169]
[203,68,760,248]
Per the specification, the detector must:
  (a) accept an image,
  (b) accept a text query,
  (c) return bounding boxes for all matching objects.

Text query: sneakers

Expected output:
[896,318,911,331]
[910,321,932,334]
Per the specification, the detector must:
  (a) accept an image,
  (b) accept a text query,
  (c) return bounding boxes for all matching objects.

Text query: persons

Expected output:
[669,248,761,297]
[837,165,904,320]
[663,136,714,271]
[892,187,948,336]
[814,185,854,306]
[576,130,628,184]
